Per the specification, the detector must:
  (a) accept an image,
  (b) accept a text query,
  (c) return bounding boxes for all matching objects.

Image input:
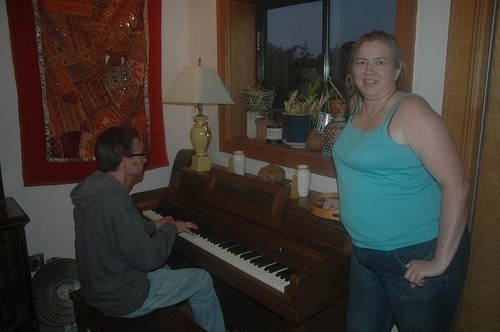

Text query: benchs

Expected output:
[69,287,208,332]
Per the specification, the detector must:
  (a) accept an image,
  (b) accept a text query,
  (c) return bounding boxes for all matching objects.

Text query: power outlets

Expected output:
[29,252,45,272]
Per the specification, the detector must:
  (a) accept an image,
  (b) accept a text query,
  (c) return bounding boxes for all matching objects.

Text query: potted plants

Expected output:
[281,74,335,149]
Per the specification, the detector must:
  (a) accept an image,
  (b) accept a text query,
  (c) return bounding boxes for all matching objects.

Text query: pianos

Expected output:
[130,148,353,332]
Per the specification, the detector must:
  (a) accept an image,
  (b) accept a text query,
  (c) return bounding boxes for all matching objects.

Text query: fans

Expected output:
[30,256,90,332]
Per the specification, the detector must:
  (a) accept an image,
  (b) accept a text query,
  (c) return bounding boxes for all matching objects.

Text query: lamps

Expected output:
[162,57,236,173]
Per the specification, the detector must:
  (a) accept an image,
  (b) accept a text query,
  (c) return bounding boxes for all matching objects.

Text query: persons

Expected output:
[331,30,471,332]
[70,127,226,332]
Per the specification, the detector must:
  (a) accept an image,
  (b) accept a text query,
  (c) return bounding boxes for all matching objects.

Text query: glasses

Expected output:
[127,147,145,158]
